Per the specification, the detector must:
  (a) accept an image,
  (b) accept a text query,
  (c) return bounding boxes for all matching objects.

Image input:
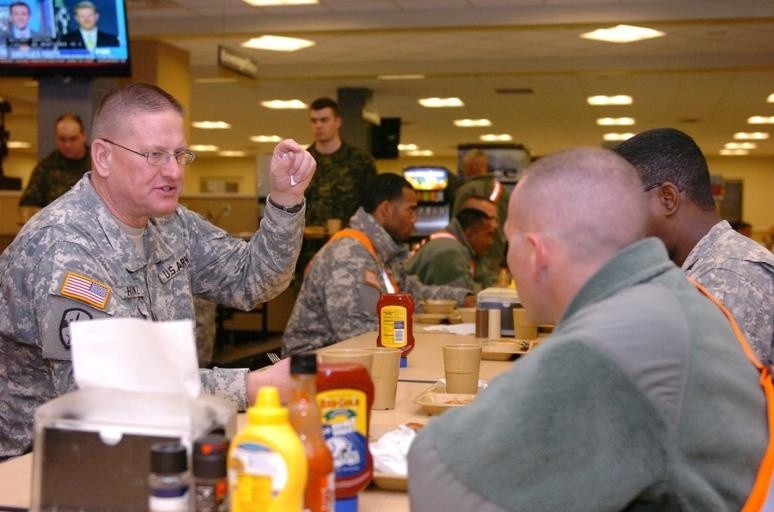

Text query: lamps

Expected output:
[241,36,310,55]
[581,23,658,47]
[259,100,308,112]
[419,98,464,107]
[598,115,636,126]
[587,93,632,108]
[601,133,637,142]
[747,116,773,124]
[452,120,491,128]
[244,136,281,145]
[733,132,769,140]
[192,121,229,132]
[184,144,218,155]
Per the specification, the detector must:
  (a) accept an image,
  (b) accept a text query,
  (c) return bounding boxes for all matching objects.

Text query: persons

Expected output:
[401,207,493,294]
[0,2,53,50]
[60,1,119,48]
[19,111,91,207]
[282,172,479,356]
[453,148,511,239]
[287,96,378,295]
[463,194,510,288]
[1,83,317,460]
[408,143,774,512]
[615,129,774,375]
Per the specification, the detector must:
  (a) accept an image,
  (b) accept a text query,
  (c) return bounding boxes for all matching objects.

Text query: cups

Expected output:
[512,308,536,339]
[322,348,372,377]
[443,344,481,393]
[370,347,401,409]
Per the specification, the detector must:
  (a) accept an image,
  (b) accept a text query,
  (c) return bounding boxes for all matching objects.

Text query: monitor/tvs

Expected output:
[0,0,131,78]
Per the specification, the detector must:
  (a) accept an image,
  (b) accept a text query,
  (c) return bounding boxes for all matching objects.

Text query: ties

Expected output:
[19,31,25,41]
[86,33,94,50]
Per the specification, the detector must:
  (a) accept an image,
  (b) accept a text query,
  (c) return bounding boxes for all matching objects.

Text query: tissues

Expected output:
[29,316,239,511]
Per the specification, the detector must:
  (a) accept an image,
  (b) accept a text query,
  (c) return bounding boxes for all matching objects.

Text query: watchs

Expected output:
[269,194,304,214]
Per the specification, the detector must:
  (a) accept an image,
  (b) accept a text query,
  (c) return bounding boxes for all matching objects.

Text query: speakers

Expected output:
[370,117,400,159]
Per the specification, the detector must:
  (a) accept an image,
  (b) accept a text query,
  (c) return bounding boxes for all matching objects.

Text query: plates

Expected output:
[416,310,462,323]
[369,423,433,490]
[479,338,528,364]
[413,379,494,414]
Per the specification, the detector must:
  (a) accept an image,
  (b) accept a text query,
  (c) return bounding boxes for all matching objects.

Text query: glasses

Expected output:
[98,136,196,166]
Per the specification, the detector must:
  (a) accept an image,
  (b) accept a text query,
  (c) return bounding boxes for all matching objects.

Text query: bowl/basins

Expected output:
[421,299,457,313]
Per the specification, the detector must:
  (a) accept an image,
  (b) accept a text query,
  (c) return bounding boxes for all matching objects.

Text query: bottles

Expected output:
[285,352,337,512]
[315,362,374,512]
[146,442,191,511]
[227,386,309,511]
[192,435,229,511]
[376,293,415,367]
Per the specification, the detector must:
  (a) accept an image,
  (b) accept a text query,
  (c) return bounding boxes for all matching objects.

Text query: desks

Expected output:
[177,192,257,236]
